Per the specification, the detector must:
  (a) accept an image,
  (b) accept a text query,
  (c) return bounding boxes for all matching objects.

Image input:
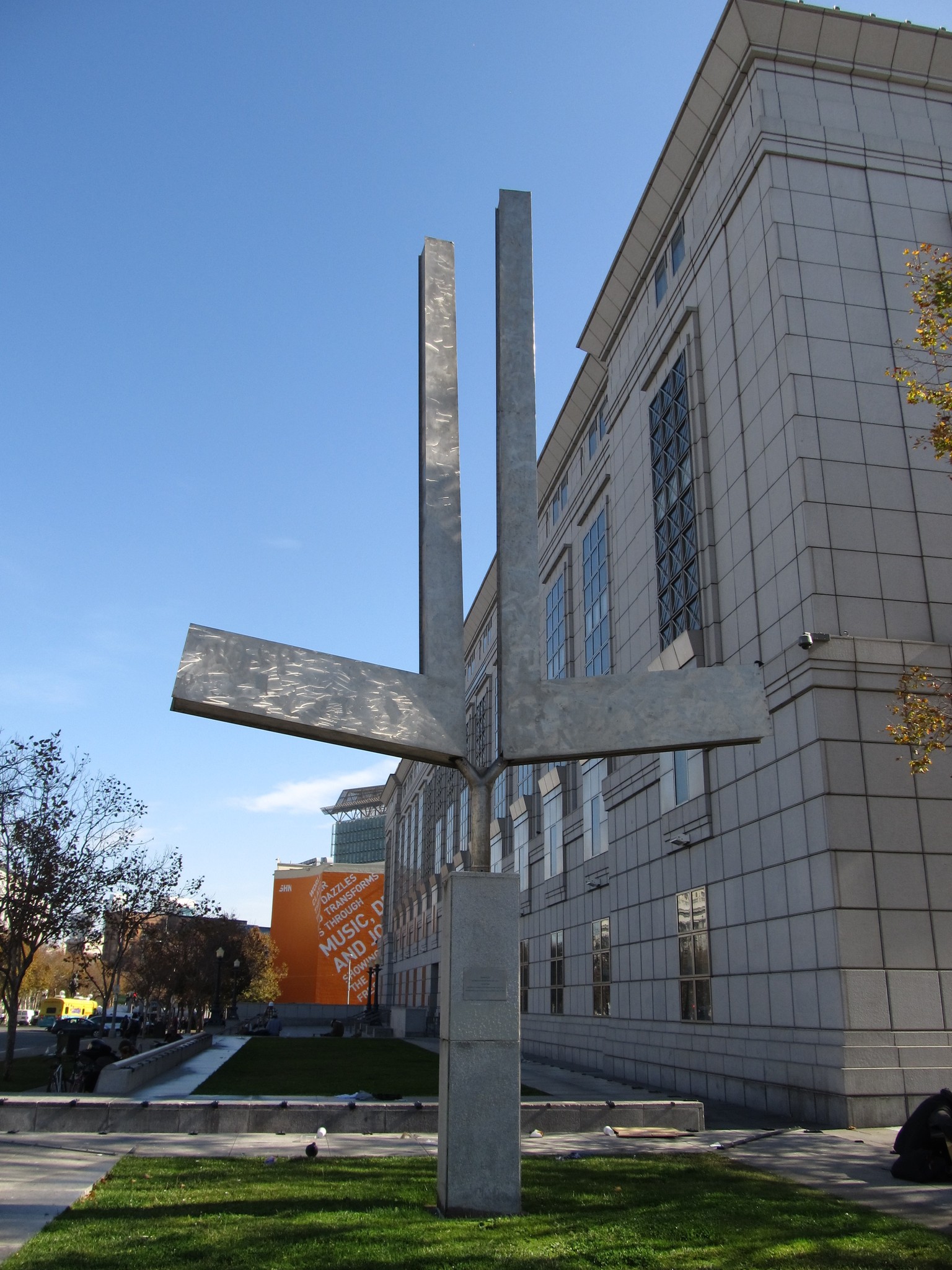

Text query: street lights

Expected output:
[231,958,240,1010]
[213,946,225,1024]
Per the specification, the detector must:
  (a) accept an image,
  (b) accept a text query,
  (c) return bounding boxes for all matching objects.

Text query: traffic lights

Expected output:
[131,993,136,1004]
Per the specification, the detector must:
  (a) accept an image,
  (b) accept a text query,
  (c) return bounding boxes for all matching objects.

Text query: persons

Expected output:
[81,1001,283,1093]
[319,1019,344,1037]
[900,1088,952,1184]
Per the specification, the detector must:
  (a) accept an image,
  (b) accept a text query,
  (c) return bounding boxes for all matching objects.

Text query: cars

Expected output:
[141,1013,157,1028]
[51,1018,106,1038]
[81,1015,130,1037]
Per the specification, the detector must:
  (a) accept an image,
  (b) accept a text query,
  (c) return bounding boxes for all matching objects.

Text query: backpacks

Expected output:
[130,1018,139,1032]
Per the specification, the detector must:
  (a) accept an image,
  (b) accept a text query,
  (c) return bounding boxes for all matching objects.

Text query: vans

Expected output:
[16,1009,40,1023]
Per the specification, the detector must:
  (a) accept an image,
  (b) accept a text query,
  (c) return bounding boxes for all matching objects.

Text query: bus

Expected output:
[37,996,98,1032]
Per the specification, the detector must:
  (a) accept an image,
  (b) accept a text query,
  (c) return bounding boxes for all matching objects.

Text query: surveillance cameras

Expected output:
[798,635,813,650]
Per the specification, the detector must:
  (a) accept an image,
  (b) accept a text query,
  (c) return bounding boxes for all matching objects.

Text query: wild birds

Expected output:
[306,1142,319,1158]
